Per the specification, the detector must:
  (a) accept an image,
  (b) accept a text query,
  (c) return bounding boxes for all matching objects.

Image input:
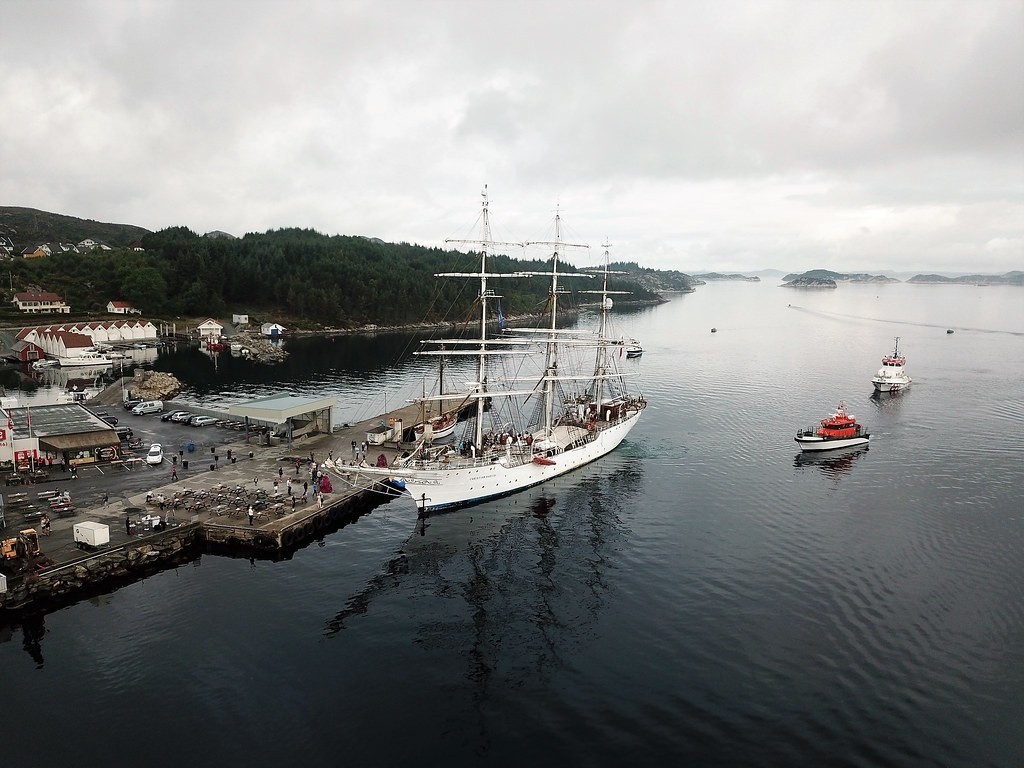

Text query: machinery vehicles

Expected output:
[0,528,41,561]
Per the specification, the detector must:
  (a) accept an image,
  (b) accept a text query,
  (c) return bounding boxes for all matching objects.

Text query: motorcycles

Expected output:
[127,437,144,449]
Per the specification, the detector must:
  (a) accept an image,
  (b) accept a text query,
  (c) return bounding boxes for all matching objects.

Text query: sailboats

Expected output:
[321,182,649,518]
[409,337,459,442]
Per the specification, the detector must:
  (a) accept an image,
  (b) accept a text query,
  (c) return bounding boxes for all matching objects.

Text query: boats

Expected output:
[870,336,913,393]
[626,345,643,359]
[794,400,871,452]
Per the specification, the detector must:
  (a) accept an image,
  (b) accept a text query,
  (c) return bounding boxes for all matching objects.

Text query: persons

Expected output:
[126,517,131,535]
[171,466,179,481]
[244,439,402,526]
[246,432,250,443]
[159,494,165,512]
[145,489,154,502]
[37,456,79,479]
[389,417,394,428]
[41,515,52,536]
[439,430,533,454]
[55,487,71,503]
[101,492,110,510]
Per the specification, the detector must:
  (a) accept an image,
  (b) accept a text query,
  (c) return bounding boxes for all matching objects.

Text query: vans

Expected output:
[131,400,164,416]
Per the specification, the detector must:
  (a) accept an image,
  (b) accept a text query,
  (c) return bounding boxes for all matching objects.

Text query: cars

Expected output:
[124,399,142,410]
[102,415,119,425]
[190,415,221,428]
[116,427,134,441]
[146,443,164,465]
[159,409,201,426]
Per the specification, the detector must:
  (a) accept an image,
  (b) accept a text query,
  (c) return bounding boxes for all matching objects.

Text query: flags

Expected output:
[496,298,504,329]
[7,411,14,430]
[25,407,31,429]
